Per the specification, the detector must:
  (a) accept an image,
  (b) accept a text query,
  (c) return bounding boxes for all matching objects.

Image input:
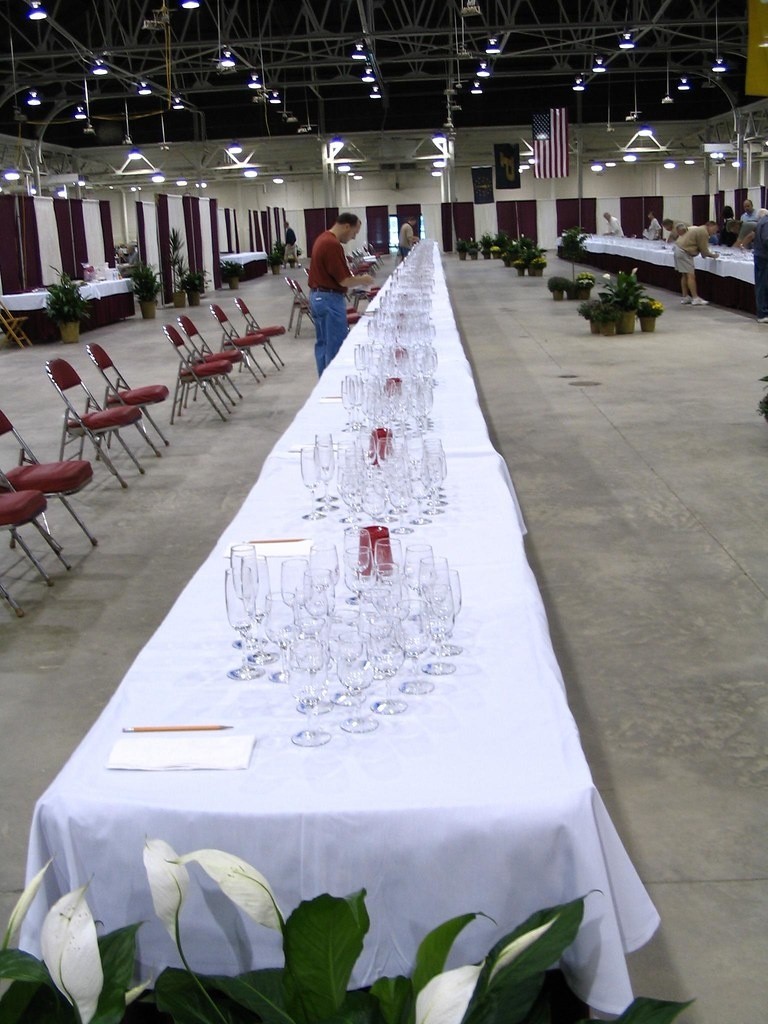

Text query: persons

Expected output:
[308,212,374,379]
[119,244,139,278]
[648,211,661,240]
[754,209,768,323]
[400,216,419,257]
[662,218,697,244]
[604,213,624,238]
[718,199,767,250]
[674,221,719,306]
[281,222,301,269]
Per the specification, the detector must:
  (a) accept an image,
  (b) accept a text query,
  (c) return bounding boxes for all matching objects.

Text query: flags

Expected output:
[494,143,521,189]
[471,165,494,204]
[532,106,570,179]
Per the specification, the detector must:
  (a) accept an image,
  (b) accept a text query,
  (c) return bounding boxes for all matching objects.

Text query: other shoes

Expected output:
[757,317,768,323]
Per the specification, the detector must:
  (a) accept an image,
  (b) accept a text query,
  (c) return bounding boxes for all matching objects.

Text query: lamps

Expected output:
[5,0,727,199]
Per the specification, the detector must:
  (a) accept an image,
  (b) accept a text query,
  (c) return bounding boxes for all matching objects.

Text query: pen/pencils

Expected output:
[322,397,344,399]
[122,725,233,734]
[247,538,307,544]
[288,450,337,453]
[365,311,374,313]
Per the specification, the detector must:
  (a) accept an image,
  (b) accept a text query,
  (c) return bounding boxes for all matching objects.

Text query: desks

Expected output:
[556,234,757,314]
[0,277,136,346]
[222,252,267,283]
[19,233,660,1021]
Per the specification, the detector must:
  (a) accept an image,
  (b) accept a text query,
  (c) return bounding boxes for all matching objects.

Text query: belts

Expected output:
[314,287,343,294]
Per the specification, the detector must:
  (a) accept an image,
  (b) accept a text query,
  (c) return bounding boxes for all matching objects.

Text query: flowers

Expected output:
[636,301,664,323]
[501,253,509,262]
[0,836,696,1024]
[490,245,501,254]
[577,301,602,322]
[576,272,595,290]
[512,259,526,269]
[528,258,547,270]
[597,268,655,313]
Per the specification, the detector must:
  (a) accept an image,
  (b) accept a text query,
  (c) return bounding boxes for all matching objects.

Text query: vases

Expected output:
[639,317,656,332]
[517,269,524,276]
[492,253,500,259]
[534,270,542,277]
[576,289,590,300]
[615,310,638,334]
[505,261,510,267]
[590,320,600,333]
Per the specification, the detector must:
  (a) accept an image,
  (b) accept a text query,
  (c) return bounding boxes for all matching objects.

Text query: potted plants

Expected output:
[467,242,482,260]
[41,265,92,344]
[166,227,191,308]
[122,253,168,319]
[516,238,548,276]
[268,248,283,275]
[181,268,212,306]
[480,233,494,259]
[556,226,592,300]
[456,233,468,260]
[220,259,244,289]
[547,277,570,301]
[508,245,520,267]
[597,303,622,336]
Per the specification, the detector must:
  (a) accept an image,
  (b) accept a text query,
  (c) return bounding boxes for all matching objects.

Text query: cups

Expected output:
[356,526,394,576]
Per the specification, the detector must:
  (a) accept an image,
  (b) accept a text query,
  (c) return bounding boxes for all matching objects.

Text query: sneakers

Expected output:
[680,294,709,306]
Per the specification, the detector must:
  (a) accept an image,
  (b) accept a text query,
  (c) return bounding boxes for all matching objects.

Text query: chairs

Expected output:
[0,241,384,618]
[0,301,34,348]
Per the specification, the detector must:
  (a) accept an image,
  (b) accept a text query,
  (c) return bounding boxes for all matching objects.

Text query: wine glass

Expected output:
[367,239,437,347]
[298,422,448,535]
[341,371,433,439]
[225,527,462,747]
[353,344,437,389]
